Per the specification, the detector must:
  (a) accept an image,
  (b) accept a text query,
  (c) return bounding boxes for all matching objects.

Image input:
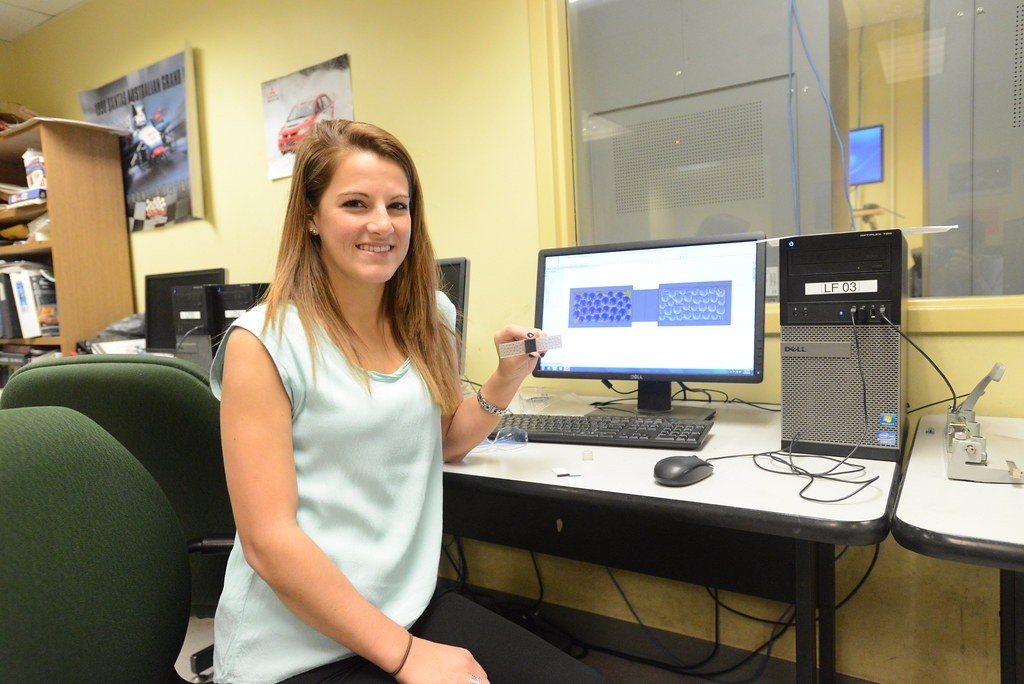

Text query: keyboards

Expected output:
[487,413,715,450]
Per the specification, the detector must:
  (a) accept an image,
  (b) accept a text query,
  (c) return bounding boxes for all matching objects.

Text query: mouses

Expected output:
[654,455,714,487]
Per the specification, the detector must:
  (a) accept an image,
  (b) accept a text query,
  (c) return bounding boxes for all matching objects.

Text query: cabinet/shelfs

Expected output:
[0,120,137,388]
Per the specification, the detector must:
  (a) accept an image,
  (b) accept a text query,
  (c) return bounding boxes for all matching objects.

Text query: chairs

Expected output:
[0,406,234,684]
[0,351,234,673]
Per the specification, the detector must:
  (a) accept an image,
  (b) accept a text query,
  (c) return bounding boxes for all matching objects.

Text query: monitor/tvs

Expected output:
[434,256,469,379]
[849,123,884,185]
[145,267,226,354]
[530,231,766,420]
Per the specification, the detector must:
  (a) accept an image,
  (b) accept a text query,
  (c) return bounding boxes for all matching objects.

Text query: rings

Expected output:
[468,675,482,684]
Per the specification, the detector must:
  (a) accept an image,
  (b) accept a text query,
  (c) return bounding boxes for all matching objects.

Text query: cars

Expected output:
[277,93,334,153]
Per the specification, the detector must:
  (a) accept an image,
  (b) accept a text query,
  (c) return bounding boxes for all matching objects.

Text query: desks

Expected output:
[890,414,1024,684]
[445,392,909,684]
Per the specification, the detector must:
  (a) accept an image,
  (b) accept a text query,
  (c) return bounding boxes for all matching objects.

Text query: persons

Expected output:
[209,117,605,684]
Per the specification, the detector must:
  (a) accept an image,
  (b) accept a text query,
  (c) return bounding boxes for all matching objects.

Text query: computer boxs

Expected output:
[173,282,271,373]
[777,229,909,461]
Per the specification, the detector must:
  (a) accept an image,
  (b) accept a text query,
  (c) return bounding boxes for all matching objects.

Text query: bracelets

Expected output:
[477,387,509,416]
[390,633,413,677]
